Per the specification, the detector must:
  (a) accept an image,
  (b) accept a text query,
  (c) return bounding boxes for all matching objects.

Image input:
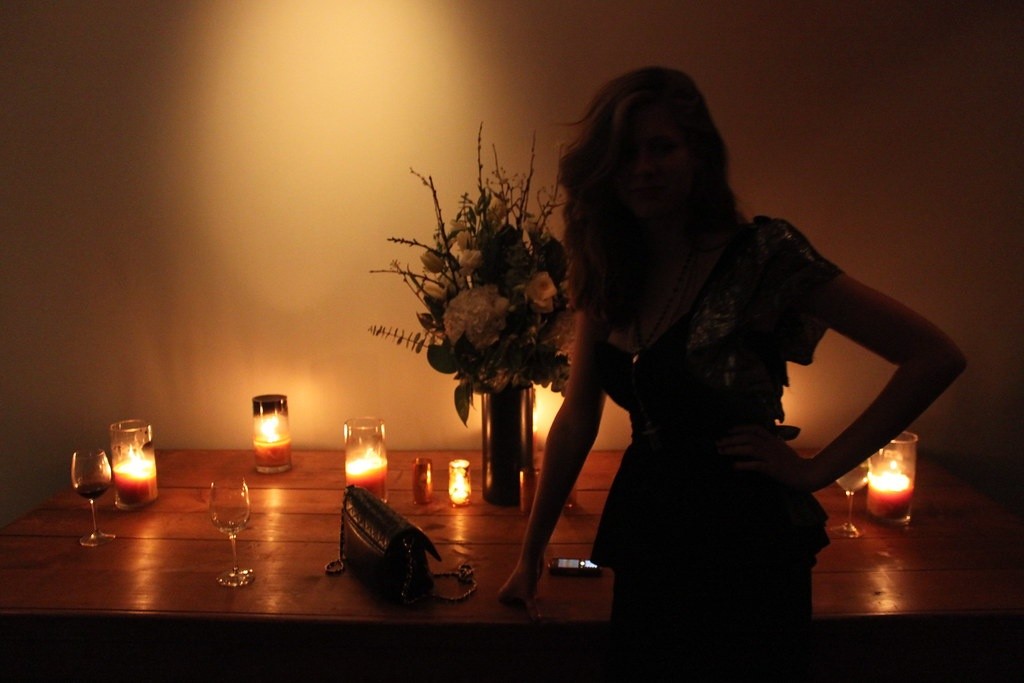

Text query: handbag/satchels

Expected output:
[324,484,478,609]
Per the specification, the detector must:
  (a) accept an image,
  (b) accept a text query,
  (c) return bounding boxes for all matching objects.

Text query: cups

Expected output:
[411,458,432,503]
[519,468,540,514]
[868,432,919,526]
[253,395,292,473]
[449,459,471,506]
[110,419,158,511]
[344,418,389,507]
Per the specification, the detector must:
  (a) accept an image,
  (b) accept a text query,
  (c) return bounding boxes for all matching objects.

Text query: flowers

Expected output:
[368,122,575,428]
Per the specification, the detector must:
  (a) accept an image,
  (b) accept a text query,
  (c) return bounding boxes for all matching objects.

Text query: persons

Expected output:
[497,66,968,683]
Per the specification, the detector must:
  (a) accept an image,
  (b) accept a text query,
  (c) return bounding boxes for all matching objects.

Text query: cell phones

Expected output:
[548,557,603,576]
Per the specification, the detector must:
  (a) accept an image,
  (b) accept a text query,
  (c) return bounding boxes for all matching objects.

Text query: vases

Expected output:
[481,384,535,511]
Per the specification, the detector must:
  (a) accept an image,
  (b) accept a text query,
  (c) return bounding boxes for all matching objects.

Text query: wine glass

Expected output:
[832,459,873,538]
[70,448,116,546]
[210,480,255,587]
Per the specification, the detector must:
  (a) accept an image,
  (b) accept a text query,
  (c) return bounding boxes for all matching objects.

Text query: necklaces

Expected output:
[626,227,699,352]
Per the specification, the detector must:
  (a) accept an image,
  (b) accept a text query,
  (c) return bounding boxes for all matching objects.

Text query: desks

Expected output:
[0,448,1024,683]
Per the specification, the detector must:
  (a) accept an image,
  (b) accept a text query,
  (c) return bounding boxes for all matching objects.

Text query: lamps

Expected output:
[866,461,916,526]
[109,444,158,512]
[343,445,388,506]
[250,416,293,474]
